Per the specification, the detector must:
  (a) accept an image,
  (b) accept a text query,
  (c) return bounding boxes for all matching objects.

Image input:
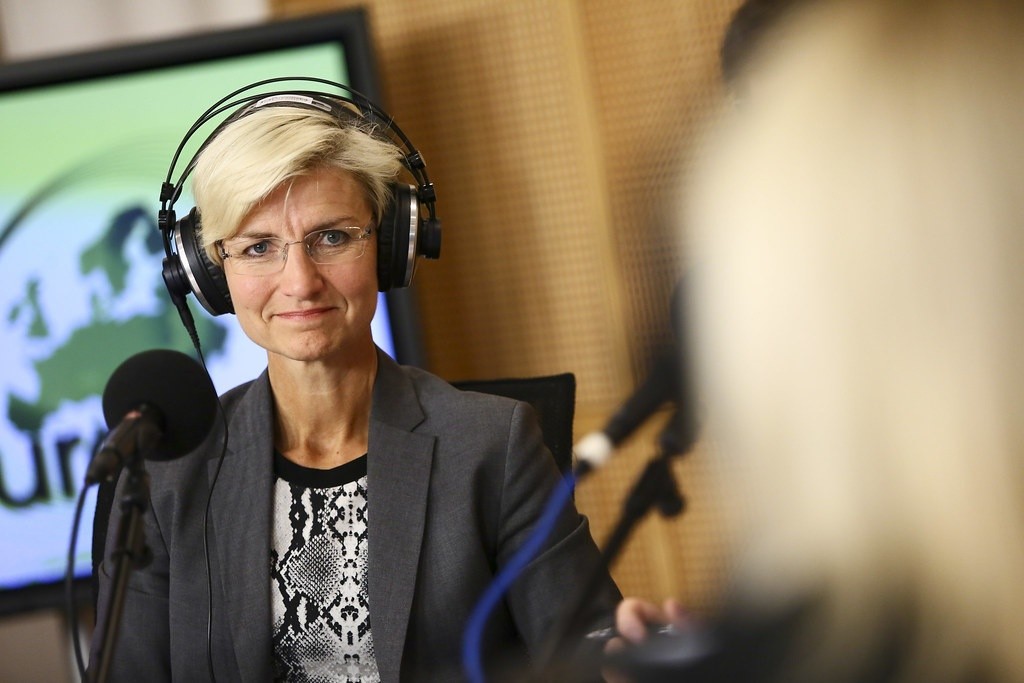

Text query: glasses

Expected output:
[215,212,378,276]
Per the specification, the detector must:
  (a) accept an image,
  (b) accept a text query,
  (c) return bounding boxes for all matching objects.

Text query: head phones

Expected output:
[161,76,440,317]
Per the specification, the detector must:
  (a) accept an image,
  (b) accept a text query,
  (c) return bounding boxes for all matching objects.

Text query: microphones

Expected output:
[86,349,217,486]
[576,297,684,468]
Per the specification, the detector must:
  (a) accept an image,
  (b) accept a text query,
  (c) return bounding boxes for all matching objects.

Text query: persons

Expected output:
[86,93,624,683]
